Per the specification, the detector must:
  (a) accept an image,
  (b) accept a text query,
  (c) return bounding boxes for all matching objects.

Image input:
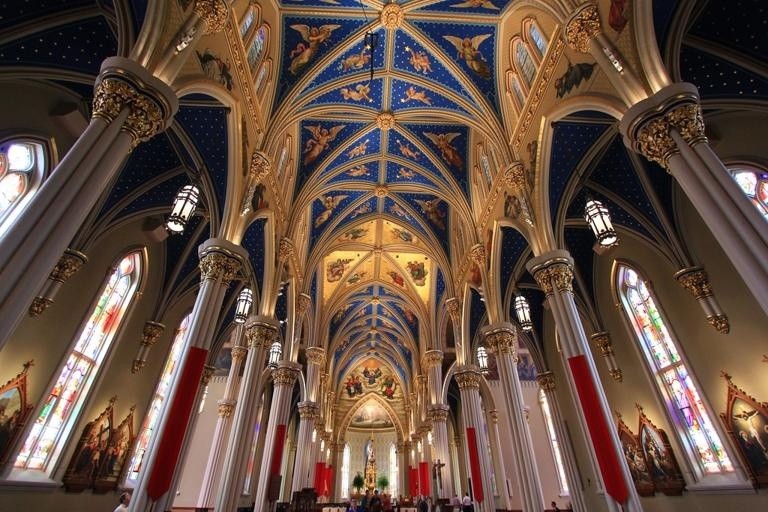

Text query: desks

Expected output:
[317,502,350,512]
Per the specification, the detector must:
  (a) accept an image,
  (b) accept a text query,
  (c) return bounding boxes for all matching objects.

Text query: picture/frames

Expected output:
[0,358,35,470]
[92,405,136,493]
[634,402,687,497]
[63,394,118,491]
[614,409,656,497]
[720,368,768,489]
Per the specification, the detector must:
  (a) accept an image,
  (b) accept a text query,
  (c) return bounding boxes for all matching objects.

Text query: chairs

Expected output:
[396,503,419,512]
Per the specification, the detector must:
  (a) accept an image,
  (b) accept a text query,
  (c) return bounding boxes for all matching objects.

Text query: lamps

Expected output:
[551,122,621,249]
[268,282,290,370]
[234,217,268,325]
[163,105,232,238]
[500,226,535,335]
[475,332,489,375]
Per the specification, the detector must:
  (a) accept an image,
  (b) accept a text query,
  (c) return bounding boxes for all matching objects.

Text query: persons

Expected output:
[452,495,462,512]
[551,501,561,512]
[361,490,372,512]
[462,492,472,512]
[112,491,132,512]
[372,490,383,512]
[382,494,393,511]
